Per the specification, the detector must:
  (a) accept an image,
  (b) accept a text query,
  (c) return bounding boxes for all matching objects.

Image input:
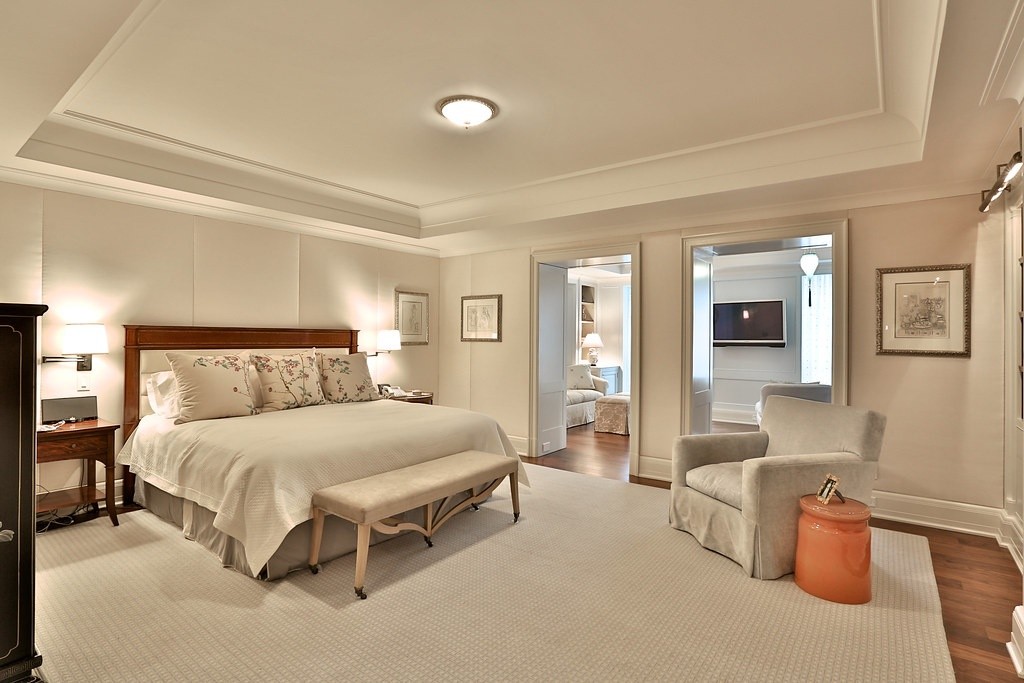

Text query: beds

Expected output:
[123,325,530,582]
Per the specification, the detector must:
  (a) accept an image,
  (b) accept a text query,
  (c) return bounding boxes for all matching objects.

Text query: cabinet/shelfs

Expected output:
[566,280,599,363]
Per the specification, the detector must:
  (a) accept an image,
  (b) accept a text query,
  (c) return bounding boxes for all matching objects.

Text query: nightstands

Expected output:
[393,390,435,404]
[36,417,121,530]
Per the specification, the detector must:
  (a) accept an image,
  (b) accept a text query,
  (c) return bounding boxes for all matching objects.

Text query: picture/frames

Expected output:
[874,263,970,356]
[814,473,847,505]
[462,294,503,342]
[394,290,430,345]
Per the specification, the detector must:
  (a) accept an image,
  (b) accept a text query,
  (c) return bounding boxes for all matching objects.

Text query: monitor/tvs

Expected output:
[712,299,788,347]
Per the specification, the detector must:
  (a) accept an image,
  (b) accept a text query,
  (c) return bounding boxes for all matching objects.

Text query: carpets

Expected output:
[32,462,961,683]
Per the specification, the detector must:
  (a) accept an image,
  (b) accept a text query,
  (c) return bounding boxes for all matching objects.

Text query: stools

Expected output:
[595,392,630,436]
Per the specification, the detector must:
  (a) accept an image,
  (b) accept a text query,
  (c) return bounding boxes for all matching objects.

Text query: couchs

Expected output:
[754,382,833,427]
[566,367,609,428]
[670,396,887,580]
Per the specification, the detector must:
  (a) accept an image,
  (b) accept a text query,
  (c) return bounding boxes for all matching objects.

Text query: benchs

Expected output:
[308,449,524,600]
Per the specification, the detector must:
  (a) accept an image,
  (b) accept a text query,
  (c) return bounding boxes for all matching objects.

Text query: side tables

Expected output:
[591,364,621,395]
[795,493,873,604]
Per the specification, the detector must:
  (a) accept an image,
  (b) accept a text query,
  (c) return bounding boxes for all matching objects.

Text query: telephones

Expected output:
[382,386,406,398]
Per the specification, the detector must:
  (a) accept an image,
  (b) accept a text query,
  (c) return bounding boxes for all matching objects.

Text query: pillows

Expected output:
[568,362,595,389]
[141,347,381,424]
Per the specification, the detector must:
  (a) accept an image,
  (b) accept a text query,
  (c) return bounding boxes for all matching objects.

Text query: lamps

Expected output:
[42,320,110,371]
[438,95,495,129]
[801,238,819,306]
[979,129,1024,213]
[583,333,604,365]
[367,330,403,357]
